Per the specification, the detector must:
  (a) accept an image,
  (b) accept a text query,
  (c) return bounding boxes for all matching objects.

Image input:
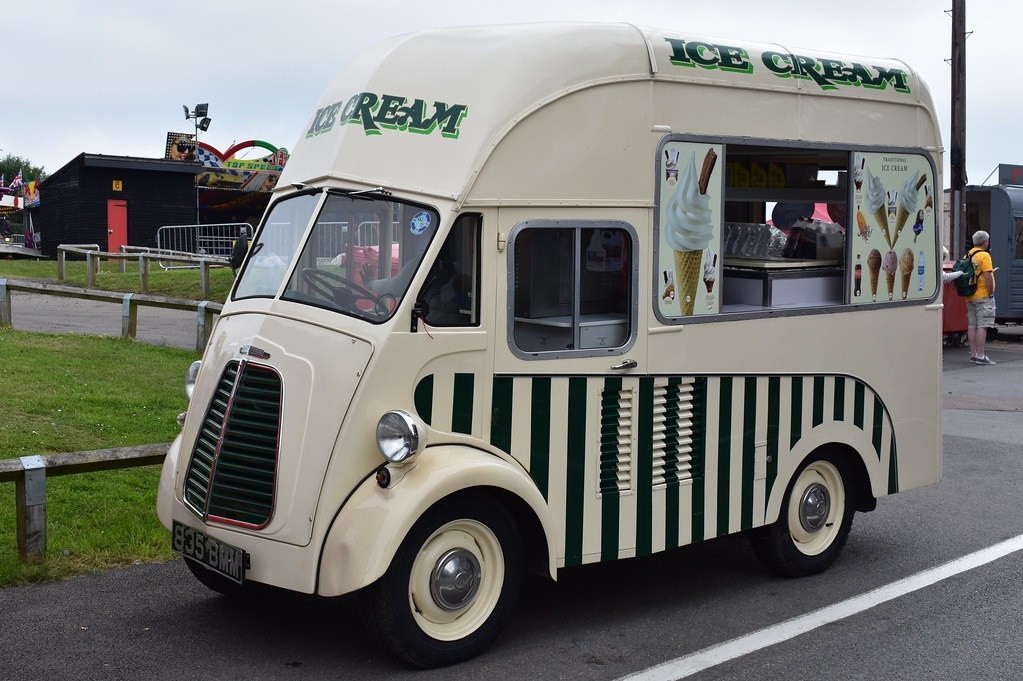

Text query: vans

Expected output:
[157,17,945,670]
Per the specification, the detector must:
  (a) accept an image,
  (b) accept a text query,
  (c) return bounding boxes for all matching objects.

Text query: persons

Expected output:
[170,134,196,160]
[260,175,279,191]
[368,225,472,327]
[943,230,996,365]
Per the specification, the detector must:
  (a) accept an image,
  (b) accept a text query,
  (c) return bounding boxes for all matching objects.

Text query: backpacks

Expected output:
[952,249,986,296]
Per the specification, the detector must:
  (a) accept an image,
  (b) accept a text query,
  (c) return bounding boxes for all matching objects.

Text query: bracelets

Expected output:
[989,294,994,298]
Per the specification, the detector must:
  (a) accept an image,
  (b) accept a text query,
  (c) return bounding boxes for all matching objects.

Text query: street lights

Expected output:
[184,103,211,252]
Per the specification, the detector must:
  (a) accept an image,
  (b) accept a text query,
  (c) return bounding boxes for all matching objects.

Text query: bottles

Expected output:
[853,254,862,297]
[781,217,823,260]
[724,222,786,257]
[917,251,925,291]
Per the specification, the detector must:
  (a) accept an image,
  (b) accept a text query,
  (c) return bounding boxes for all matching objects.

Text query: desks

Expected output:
[458,309,627,352]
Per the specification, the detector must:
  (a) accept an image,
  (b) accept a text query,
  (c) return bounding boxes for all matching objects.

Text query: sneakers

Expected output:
[975,356,996,365]
[969,357,977,363]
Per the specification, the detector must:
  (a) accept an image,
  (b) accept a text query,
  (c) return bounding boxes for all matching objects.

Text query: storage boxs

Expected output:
[817,232,847,261]
[725,162,818,188]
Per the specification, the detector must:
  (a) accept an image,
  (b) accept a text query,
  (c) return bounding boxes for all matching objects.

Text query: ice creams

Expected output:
[853,152,933,248]
[663,147,717,316]
[867,248,915,302]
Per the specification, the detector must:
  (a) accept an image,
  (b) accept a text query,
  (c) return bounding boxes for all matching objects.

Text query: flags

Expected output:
[0,169,22,189]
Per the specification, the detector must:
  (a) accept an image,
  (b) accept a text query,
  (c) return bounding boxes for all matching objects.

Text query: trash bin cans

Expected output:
[340,242,400,312]
